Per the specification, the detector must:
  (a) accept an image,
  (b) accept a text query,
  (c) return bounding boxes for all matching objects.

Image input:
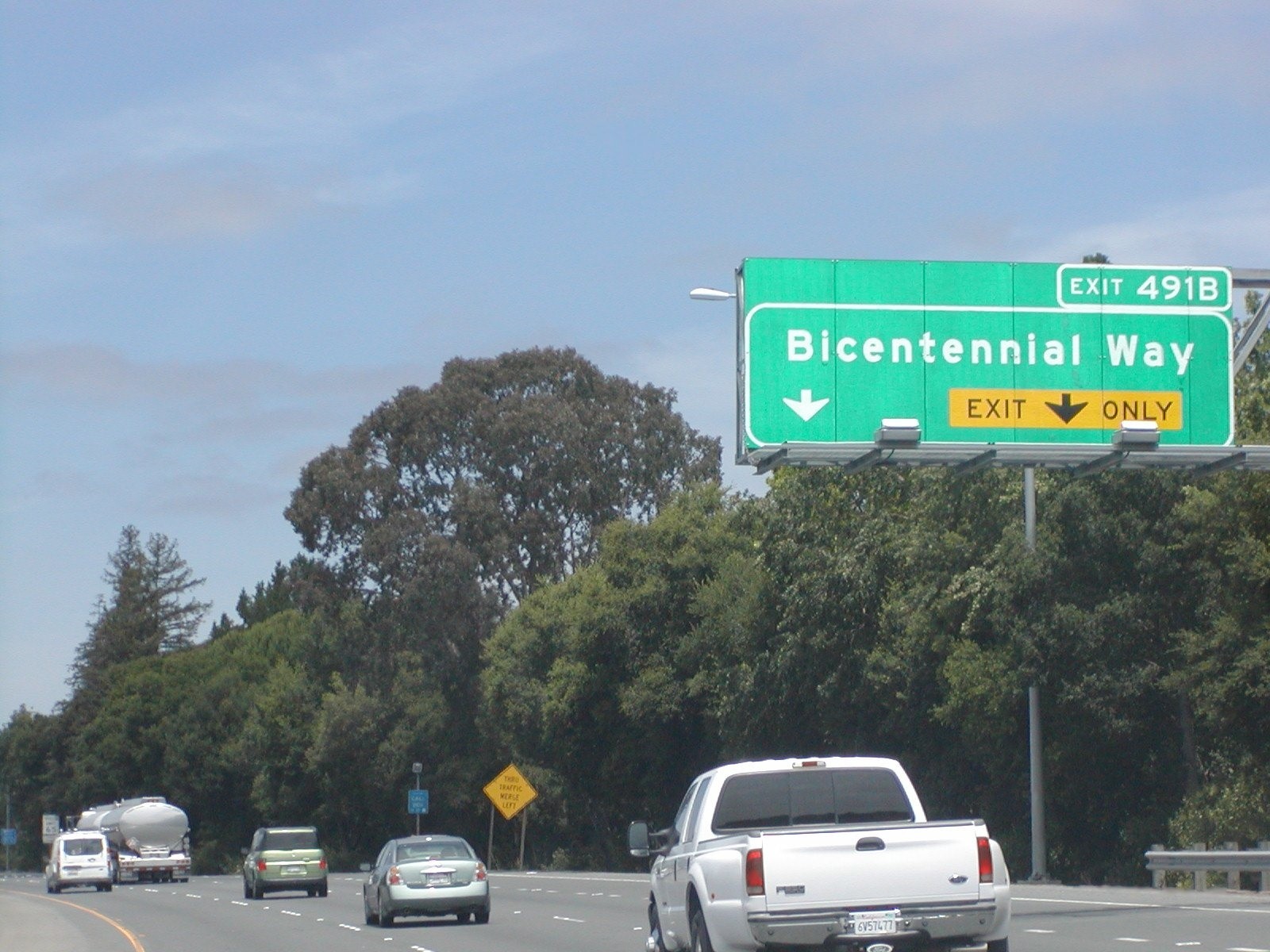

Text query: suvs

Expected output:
[241,826,329,900]
[45,833,112,892]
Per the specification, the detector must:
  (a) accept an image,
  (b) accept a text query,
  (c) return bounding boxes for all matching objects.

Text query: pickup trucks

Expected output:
[625,758,1011,951]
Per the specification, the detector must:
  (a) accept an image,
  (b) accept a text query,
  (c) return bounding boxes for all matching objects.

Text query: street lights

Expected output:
[691,287,739,303]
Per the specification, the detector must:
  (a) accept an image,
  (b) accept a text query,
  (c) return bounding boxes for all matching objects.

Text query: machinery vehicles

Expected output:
[67,795,191,883]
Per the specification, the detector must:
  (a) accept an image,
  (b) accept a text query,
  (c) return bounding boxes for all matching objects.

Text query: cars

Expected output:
[364,835,491,923]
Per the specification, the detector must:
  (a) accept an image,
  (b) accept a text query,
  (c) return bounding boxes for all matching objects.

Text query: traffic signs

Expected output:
[741,257,1233,454]
